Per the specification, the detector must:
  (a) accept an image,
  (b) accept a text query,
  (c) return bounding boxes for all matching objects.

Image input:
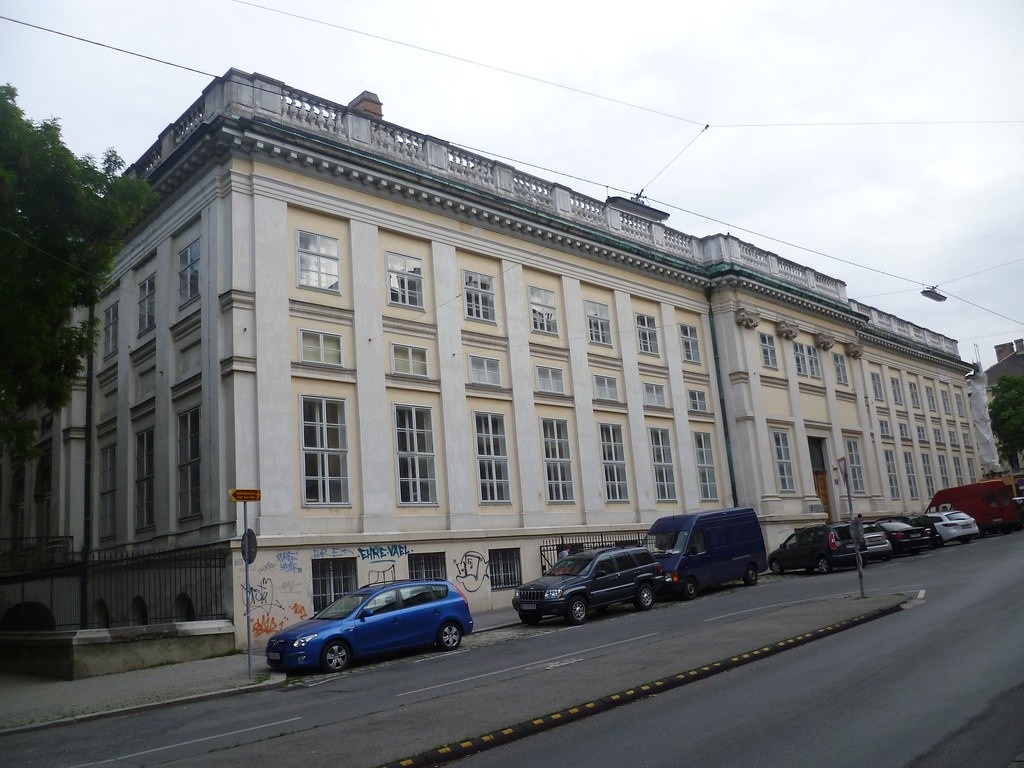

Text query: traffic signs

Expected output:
[229,487,261,504]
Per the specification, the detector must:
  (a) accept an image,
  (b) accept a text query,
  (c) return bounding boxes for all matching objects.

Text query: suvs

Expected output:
[511,544,665,627]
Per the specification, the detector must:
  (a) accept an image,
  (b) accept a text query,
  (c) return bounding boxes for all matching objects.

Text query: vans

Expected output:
[923,479,1024,539]
[632,506,768,602]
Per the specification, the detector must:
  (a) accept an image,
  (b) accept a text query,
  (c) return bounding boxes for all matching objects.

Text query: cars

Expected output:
[909,511,980,545]
[859,519,893,562]
[265,577,475,674]
[875,516,932,556]
[767,520,857,576]
